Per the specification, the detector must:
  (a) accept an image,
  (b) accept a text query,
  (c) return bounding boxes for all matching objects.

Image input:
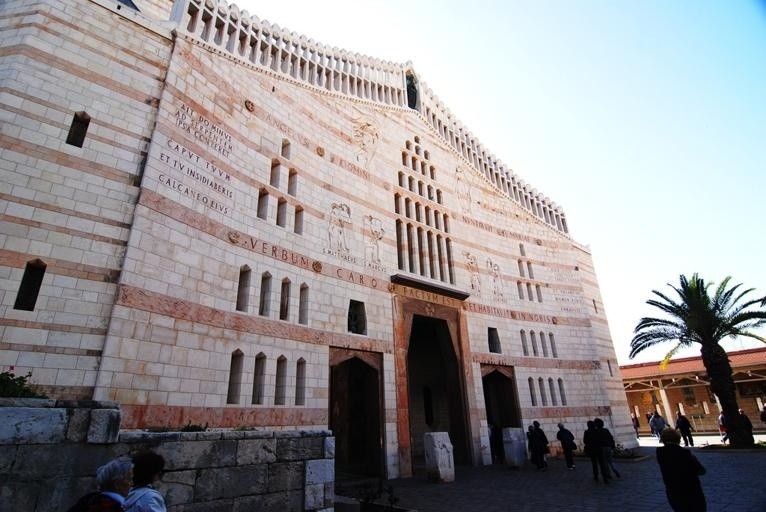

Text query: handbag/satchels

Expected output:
[573,442,577,450]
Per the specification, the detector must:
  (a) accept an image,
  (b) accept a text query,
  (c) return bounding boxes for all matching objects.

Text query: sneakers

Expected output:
[535,464,545,471]
[567,464,578,471]
[593,468,621,485]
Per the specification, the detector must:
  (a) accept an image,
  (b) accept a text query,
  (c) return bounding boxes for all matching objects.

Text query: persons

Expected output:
[673,410,697,448]
[649,409,672,444]
[631,412,657,441]
[527,425,550,467]
[657,426,709,511]
[594,418,621,480]
[718,410,732,446]
[583,419,611,484]
[122,448,168,512]
[739,408,754,445]
[557,422,577,471]
[528,420,548,472]
[67,458,137,512]
